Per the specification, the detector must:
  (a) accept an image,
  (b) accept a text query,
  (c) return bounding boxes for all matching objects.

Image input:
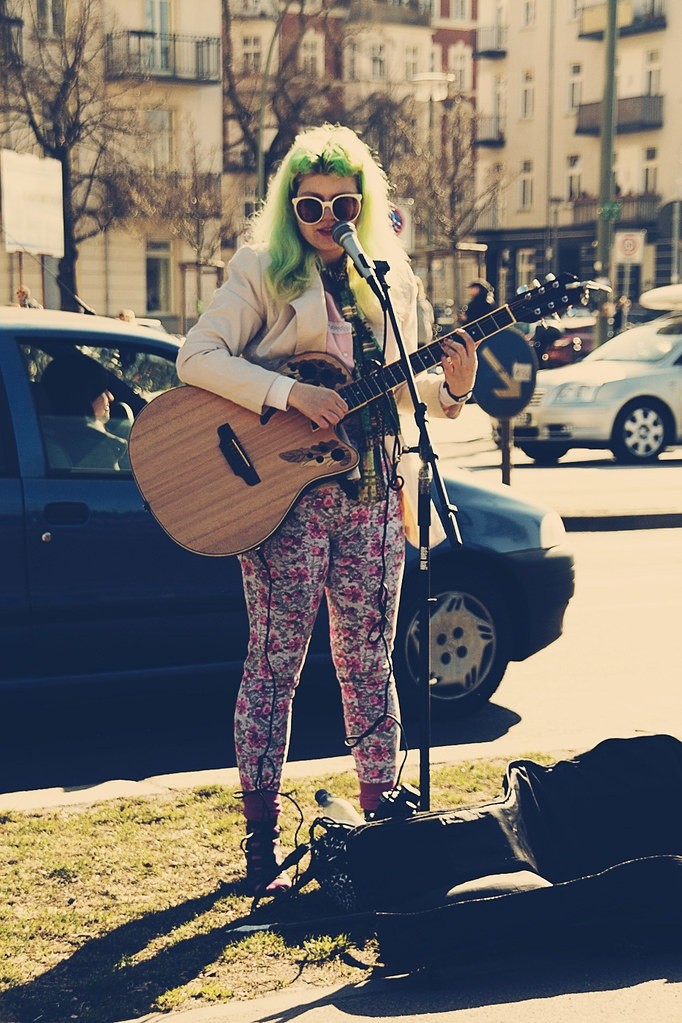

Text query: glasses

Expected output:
[291,195,364,226]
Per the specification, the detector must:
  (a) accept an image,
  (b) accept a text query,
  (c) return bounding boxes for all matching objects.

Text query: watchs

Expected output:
[443,381,473,402]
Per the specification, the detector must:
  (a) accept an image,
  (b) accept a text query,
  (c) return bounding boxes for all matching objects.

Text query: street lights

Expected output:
[447,48,508,319]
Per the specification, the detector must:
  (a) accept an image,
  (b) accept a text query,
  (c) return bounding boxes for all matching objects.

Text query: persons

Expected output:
[39,354,128,486]
[16,285,43,310]
[460,278,495,325]
[176,122,481,895]
[415,276,435,348]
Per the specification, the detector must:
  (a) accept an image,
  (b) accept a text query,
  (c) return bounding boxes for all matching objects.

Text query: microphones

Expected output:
[331,221,388,311]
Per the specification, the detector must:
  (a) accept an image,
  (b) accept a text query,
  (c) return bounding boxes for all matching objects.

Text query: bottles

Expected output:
[315,790,368,831]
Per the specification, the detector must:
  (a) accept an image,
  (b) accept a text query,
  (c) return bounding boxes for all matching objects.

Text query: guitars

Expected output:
[126,270,613,559]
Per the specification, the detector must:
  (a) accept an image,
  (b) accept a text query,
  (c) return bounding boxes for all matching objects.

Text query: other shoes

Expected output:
[246,822,292,896]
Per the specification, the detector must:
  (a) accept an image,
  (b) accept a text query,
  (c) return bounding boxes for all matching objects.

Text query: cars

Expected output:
[0,305,577,720]
[489,282,682,466]
